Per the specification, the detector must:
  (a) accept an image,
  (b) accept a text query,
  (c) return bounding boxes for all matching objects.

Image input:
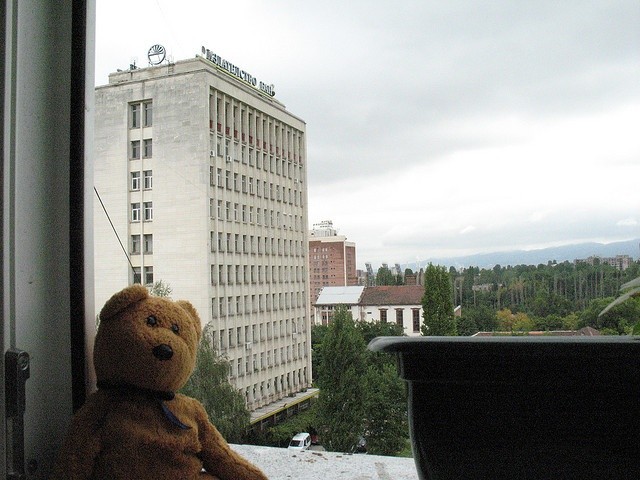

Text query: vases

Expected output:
[368,334,639,480]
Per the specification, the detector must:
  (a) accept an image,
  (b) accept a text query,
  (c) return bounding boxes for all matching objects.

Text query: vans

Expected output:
[287,433,312,450]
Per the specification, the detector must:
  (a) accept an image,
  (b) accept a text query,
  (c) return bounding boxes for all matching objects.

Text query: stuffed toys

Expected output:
[58,283,266,480]
